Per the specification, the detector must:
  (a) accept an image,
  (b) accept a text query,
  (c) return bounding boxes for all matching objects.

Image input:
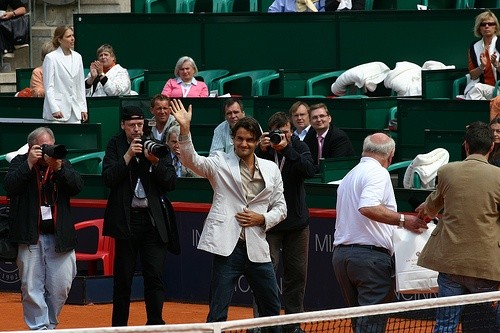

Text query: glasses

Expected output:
[481,22,496,27]
[312,114,329,120]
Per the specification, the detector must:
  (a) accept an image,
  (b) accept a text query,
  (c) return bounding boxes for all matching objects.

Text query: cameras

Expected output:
[135,135,170,158]
[36,144,68,159]
[265,130,284,144]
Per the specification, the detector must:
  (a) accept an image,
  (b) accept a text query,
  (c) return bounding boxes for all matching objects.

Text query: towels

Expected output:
[6,142,29,163]
[404,147,450,190]
[331,59,455,96]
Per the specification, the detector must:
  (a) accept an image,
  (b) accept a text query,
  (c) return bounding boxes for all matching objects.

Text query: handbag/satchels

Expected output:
[11,15,28,44]
[392,229,439,295]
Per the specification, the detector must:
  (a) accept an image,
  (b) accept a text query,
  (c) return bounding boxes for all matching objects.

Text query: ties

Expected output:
[317,137,323,164]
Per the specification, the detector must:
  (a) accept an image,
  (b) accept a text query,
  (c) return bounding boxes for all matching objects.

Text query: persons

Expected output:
[332,132,429,333]
[209,98,246,157]
[416,121,500,333]
[268,0,326,12]
[0,0,28,53]
[305,103,355,174]
[165,125,199,177]
[102,106,181,327]
[289,101,313,142]
[1,127,77,331]
[169,98,287,333]
[255,112,314,333]
[488,118,500,168]
[464,11,500,100]
[41,25,90,123]
[149,94,179,144]
[30,40,56,97]
[85,44,131,97]
[161,56,209,97]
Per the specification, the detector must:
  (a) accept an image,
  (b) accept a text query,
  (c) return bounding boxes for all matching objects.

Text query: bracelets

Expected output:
[13,11,15,16]
[400,214,405,227]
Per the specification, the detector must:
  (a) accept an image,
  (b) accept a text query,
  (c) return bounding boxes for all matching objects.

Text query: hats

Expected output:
[121,106,145,120]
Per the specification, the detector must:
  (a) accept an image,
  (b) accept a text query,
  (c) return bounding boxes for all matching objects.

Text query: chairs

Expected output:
[0,0,500,185]
[74,219,115,275]
